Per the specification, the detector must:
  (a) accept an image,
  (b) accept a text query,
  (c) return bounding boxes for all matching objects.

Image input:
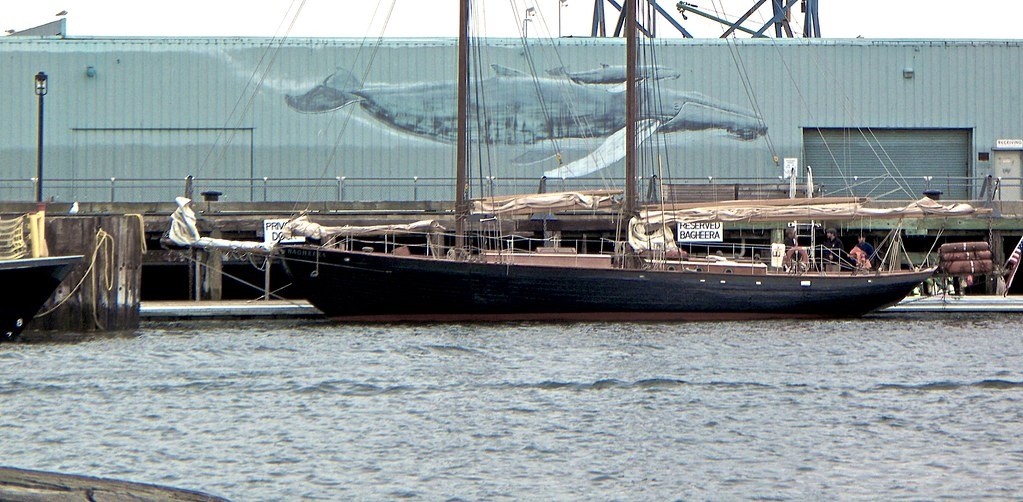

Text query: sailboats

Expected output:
[159,0,992,324]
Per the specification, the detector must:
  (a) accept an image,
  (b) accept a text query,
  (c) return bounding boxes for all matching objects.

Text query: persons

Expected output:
[784,229,796,258]
[852,234,875,266]
[824,228,843,261]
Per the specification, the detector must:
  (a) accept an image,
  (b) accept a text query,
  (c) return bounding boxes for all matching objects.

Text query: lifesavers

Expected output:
[786,247,808,273]
[724,268,734,274]
[667,265,676,271]
[695,267,704,272]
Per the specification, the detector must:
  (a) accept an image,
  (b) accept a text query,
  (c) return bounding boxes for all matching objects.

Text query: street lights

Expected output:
[33,71,48,203]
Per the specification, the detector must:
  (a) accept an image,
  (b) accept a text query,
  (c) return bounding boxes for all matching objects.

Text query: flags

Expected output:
[1004,239,1023,297]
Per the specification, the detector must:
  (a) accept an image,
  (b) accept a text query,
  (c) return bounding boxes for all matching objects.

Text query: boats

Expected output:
[0,206,85,343]
